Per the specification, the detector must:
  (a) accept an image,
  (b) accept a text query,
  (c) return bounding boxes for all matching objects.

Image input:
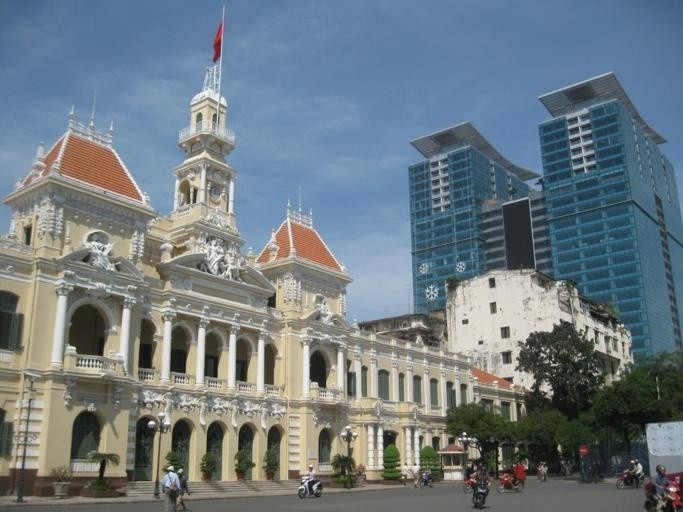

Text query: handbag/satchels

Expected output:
[169,482,176,491]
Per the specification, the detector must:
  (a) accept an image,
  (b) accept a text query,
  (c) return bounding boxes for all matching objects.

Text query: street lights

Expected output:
[145,413,173,499]
[13,367,43,506]
[457,430,473,480]
[339,424,359,488]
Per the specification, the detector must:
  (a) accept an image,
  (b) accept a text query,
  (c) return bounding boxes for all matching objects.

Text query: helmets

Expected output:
[656,464,665,472]
[481,466,486,471]
[309,465,313,468]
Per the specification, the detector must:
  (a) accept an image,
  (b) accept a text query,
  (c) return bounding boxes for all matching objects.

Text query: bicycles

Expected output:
[419,472,434,489]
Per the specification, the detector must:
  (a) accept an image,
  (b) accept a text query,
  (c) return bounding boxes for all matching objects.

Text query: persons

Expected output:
[474,457,549,494]
[629,458,669,512]
[411,463,420,488]
[177,469,190,510]
[402,462,410,486]
[162,465,181,512]
[306,464,315,495]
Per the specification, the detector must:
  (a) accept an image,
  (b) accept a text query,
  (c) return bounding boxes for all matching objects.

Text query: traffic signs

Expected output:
[579,445,588,455]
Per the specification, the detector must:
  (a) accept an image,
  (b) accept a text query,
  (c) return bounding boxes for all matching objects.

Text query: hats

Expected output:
[166,466,175,472]
[177,469,184,474]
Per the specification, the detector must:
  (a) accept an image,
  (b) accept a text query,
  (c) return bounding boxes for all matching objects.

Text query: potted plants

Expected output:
[200,454,220,481]
[331,474,355,488]
[233,449,255,480]
[262,449,280,480]
[48,464,74,499]
[356,463,367,486]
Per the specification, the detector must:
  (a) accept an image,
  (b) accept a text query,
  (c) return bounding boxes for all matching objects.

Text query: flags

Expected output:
[213,22,222,63]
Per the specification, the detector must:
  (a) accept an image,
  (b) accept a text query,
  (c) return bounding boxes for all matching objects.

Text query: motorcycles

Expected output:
[615,468,646,490]
[470,477,490,509]
[497,469,524,494]
[556,459,603,484]
[643,478,683,512]
[538,467,547,482]
[464,473,491,494]
[297,477,323,499]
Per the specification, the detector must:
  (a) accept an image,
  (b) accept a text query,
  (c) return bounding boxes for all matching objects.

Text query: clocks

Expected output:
[210,188,220,200]
